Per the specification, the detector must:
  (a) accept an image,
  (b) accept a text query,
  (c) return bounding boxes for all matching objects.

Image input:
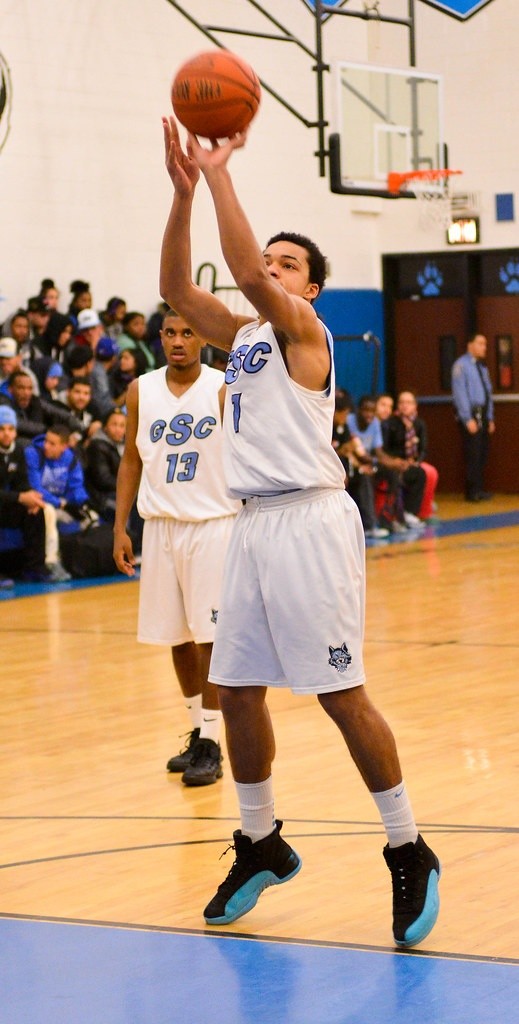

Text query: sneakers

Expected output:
[203,819,301,924]
[182,738,223,784]
[166,727,200,771]
[382,832,441,946]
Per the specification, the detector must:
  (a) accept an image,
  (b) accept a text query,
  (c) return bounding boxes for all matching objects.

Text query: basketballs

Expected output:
[173,54,261,135]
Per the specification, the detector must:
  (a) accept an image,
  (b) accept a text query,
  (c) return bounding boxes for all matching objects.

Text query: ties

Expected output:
[475,361,489,407]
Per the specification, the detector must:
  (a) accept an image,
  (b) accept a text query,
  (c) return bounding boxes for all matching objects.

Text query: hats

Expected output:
[77,308,100,329]
[0,337,18,358]
[95,338,119,360]
[0,405,16,426]
[42,361,63,377]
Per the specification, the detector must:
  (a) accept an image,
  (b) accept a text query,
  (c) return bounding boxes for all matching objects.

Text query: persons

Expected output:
[0,277,228,584]
[453,335,495,504]
[113,304,245,789]
[157,112,442,948]
[331,382,440,537]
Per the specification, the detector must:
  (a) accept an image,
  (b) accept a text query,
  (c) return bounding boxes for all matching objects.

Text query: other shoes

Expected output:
[0,564,69,586]
[363,511,426,538]
